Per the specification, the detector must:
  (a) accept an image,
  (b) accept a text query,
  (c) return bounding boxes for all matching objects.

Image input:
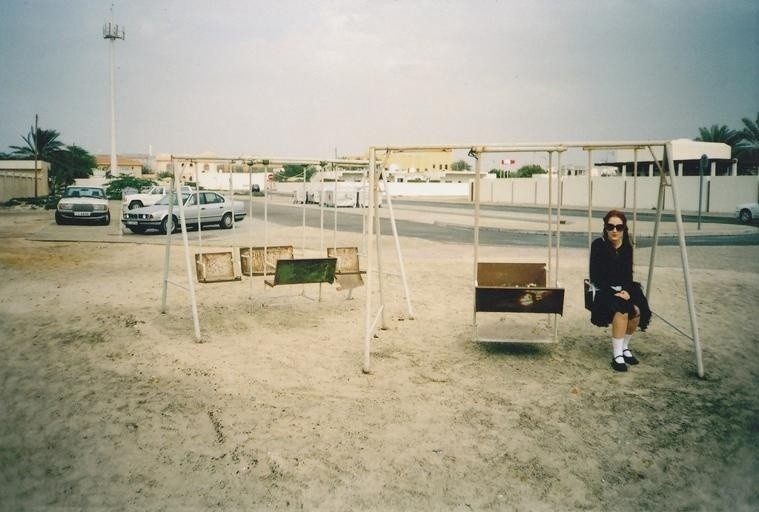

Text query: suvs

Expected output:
[122,185,204,210]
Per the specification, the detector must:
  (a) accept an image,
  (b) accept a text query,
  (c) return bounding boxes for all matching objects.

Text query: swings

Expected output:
[469,147,565,343]
[583,146,641,311]
[184,162,367,298]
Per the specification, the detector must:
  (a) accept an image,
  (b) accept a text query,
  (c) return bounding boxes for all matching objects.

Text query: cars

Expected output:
[252,184,260,193]
[55,186,110,225]
[121,190,246,234]
[736,200,759,225]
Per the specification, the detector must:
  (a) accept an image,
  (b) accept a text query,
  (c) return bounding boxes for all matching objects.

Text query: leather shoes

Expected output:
[612,355,627,371]
[623,349,639,365]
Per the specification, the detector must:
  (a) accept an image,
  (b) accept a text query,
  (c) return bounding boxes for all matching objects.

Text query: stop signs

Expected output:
[268,174,273,180]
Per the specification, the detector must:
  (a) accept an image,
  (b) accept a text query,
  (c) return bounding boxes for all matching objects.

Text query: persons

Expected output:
[590,209,642,373]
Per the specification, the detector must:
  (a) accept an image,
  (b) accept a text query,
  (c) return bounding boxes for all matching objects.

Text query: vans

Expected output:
[185,181,196,190]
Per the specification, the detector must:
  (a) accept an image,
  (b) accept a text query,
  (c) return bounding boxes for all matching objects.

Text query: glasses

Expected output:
[604,223,624,232]
